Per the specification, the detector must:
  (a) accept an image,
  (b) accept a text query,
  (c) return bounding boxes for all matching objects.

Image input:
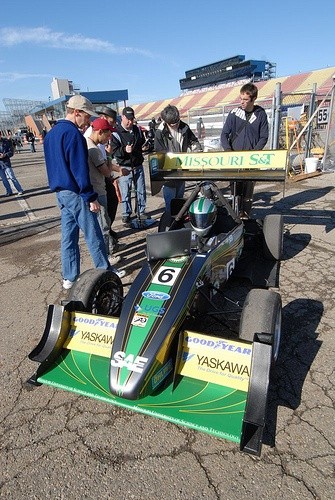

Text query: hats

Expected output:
[160,103,180,124]
[67,94,100,118]
[122,106,135,119]
[92,119,116,132]
[95,106,117,118]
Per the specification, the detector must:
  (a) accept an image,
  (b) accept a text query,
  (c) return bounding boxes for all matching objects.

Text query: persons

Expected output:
[42,126,47,144]
[11,132,23,154]
[26,128,36,153]
[148,116,162,131]
[197,117,204,139]
[221,83,269,215]
[44,95,127,289]
[188,198,227,253]
[82,105,127,266]
[153,104,204,212]
[110,107,150,228]
[0,131,25,196]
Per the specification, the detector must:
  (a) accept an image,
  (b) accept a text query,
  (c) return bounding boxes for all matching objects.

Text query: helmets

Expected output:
[189,197,218,236]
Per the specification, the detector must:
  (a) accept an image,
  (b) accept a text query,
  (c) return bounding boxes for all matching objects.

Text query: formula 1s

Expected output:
[27,149,290,458]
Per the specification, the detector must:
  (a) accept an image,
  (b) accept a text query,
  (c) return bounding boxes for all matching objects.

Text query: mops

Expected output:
[292,117,322,182]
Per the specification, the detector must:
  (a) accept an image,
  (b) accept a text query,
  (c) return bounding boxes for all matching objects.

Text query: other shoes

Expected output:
[19,192,22,194]
[116,270,126,278]
[5,192,13,197]
[108,255,121,266]
[110,243,125,253]
[62,278,80,289]
[122,216,129,223]
[137,213,151,219]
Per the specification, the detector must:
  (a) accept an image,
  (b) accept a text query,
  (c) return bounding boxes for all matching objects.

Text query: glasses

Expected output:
[170,120,180,126]
